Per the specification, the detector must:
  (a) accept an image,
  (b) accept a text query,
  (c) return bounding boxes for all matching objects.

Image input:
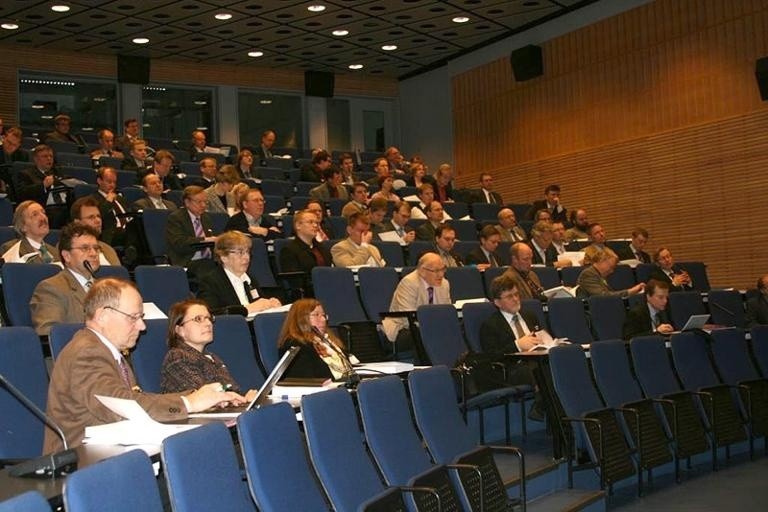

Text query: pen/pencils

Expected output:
[213,383,232,393]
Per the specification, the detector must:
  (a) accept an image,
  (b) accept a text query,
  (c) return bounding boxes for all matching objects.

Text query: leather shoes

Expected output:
[528,399,546,421]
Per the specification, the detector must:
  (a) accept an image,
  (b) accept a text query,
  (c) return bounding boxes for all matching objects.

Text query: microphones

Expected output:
[0,375,78,480]
[83,260,102,280]
[710,301,752,332]
[310,325,360,389]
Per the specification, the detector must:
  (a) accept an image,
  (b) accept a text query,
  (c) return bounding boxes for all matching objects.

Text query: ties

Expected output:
[194,218,210,258]
[41,246,52,263]
[120,360,131,388]
[112,200,126,228]
[488,254,497,267]
[428,287,433,304]
[45,171,62,204]
[511,229,519,241]
[512,315,525,337]
[489,193,495,204]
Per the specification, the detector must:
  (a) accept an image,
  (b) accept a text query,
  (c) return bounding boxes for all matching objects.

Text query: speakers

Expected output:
[117,55,151,84]
[305,70,335,96]
[510,45,544,81]
[755,57,768,101]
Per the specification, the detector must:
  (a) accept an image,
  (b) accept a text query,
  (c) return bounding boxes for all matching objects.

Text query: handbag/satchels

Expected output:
[457,350,499,399]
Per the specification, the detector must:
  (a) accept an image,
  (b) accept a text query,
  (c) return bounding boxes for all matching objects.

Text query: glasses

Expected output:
[70,245,101,253]
[181,314,216,326]
[424,265,448,273]
[226,248,251,257]
[104,306,146,322]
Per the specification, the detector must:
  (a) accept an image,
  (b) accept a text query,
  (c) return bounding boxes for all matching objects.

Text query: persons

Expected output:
[43,279,238,459]
[159,300,258,408]
[745,275,767,328]
[279,146,693,428]
[1,114,282,334]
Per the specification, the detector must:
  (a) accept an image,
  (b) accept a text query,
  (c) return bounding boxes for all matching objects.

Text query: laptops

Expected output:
[186,345,303,419]
[664,314,709,339]
